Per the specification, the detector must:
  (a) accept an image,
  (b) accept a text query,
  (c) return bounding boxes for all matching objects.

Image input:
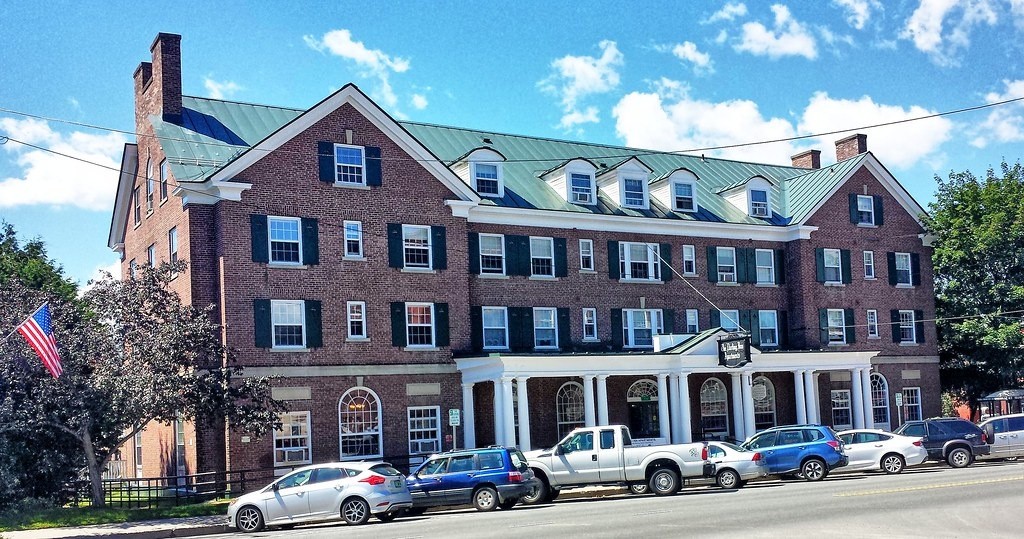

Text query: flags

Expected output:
[17,304,62,377]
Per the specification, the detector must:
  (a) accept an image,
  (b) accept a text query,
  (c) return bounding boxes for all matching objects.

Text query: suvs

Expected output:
[738,423,849,482]
[891,416,995,469]
[226,460,414,534]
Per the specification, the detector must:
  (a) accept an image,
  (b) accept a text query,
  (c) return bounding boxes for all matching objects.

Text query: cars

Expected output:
[792,429,929,480]
[974,413,1024,460]
[628,440,770,495]
[405,444,532,517]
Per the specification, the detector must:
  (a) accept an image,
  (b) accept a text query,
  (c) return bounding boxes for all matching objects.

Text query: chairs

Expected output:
[857,434,862,443]
[587,434,593,450]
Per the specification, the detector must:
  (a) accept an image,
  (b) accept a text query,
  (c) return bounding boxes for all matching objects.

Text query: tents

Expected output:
[977,389,1024,417]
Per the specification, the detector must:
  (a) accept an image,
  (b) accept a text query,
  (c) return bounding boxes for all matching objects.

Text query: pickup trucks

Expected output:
[511,425,717,506]
